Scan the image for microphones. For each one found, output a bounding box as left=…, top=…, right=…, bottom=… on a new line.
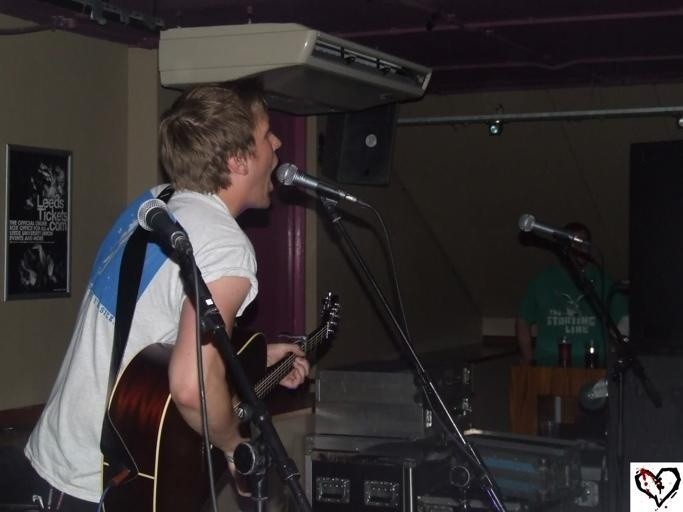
left=518, top=214, right=585, bottom=244
left=139, top=199, right=192, bottom=257
left=276, top=163, right=358, bottom=204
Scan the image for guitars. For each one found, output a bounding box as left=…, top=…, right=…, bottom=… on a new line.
left=99, top=289, right=342, bottom=511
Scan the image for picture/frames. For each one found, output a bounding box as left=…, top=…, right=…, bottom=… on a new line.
left=2, top=141, right=73, bottom=305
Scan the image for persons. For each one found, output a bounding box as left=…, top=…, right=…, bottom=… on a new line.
left=515, top=220, right=629, bottom=366
left=23, top=85, right=312, bottom=512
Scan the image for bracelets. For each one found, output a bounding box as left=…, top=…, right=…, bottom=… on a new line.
left=227, top=455, right=234, bottom=464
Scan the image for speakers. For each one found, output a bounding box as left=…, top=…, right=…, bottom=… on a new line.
left=321, top=99, right=399, bottom=187
left=609, top=355, right=683, bottom=511
left=630, top=140, right=683, bottom=355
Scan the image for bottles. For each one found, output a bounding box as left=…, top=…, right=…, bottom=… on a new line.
left=584, top=337, right=599, bottom=368
left=558, top=338, right=570, bottom=365
